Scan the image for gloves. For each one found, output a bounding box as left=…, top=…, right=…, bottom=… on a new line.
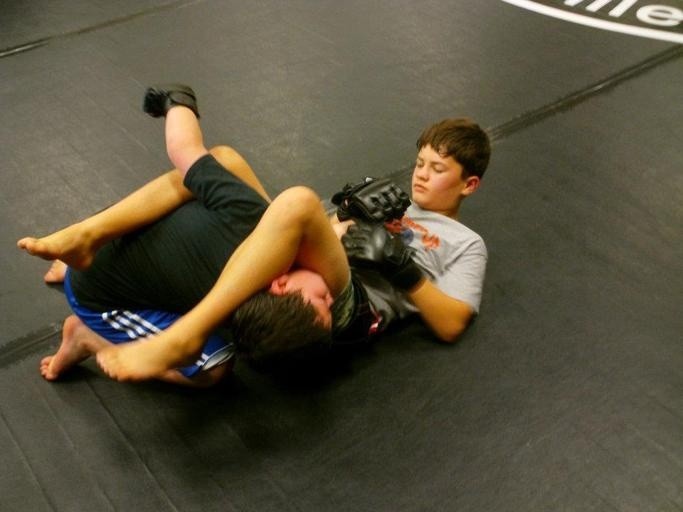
left=331, top=176, right=411, bottom=224
left=143, top=83, right=200, bottom=119
left=341, top=221, right=422, bottom=290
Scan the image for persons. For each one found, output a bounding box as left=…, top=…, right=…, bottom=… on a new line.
left=39, top=82, right=336, bottom=396
left=17, top=115, right=490, bottom=385
